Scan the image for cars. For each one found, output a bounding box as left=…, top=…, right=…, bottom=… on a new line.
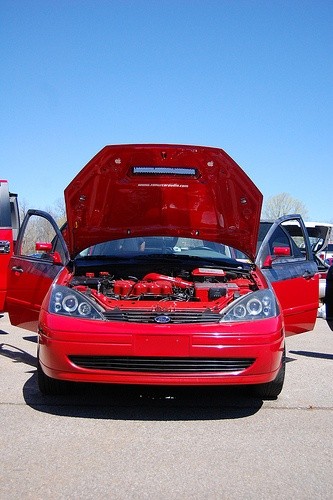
left=5, top=143, right=319, bottom=401
left=278, top=220, right=333, bottom=297
